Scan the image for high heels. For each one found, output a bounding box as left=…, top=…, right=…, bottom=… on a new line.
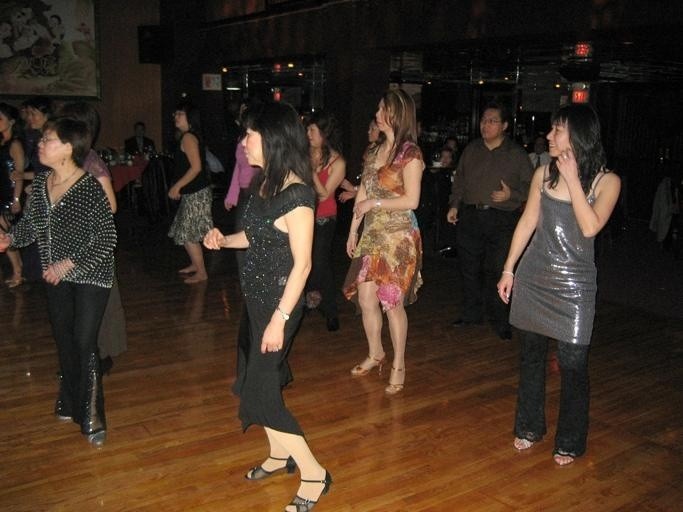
left=283, top=467, right=334, bottom=512
left=383, top=364, right=407, bottom=396
left=101, top=356, right=113, bottom=377
left=244, top=455, right=296, bottom=481
left=348, top=352, right=388, bottom=379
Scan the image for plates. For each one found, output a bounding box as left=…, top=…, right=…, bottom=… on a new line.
left=432, top=165, right=442, bottom=168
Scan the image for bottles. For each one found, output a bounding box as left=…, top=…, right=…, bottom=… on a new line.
left=516, top=123, right=528, bottom=142
left=419, top=115, right=470, bottom=146
left=665, top=148, right=670, bottom=161
left=97, top=145, right=153, bottom=168
left=656, top=147, right=665, bottom=168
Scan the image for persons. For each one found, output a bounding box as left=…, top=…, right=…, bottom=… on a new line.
left=299, top=95, right=386, bottom=332
left=160, top=100, right=262, bottom=294
left=343, top=89, right=428, bottom=395
left=1, top=117, right=117, bottom=447
left=50, top=99, right=127, bottom=377
left=0, top=99, right=50, bottom=288
left=125, top=123, right=156, bottom=207
left=497, top=105, right=621, bottom=464
left=416, top=106, right=553, bottom=342
left=204, top=99, right=332, bottom=511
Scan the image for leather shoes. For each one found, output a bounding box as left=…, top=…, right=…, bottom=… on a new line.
left=450, top=319, right=481, bottom=329
left=82, top=429, right=107, bottom=448
left=325, top=317, right=340, bottom=332
left=494, top=326, right=512, bottom=343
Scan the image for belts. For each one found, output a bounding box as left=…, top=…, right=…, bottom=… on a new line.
left=460, top=202, right=496, bottom=212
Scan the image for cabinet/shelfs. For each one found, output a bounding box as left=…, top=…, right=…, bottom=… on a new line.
left=224, top=54, right=327, bottom=138
left=391, top=41, right=579, bottom=151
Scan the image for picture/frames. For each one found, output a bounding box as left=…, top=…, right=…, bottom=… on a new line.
left=0, top=1, right=101, bottom=100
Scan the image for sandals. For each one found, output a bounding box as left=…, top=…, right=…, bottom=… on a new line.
left=552, top=450, right=576, bottom=466
left=512, top=436, right=537, bottom=451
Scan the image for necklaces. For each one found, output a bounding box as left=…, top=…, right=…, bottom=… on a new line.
left=50, top=166, right=79, bottom=193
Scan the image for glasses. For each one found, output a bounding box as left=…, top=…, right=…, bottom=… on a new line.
left=479, top=116, right=507, bottom=124
left=37, top=136, right=71, bottom=144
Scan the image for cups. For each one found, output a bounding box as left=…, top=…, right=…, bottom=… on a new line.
left=432, top=162, right=441, bottom=166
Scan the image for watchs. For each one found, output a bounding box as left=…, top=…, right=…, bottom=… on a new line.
left=277, top=307, right=291, bottom=322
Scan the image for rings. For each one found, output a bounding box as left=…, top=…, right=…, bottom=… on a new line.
left=564, top=156, right=568, bottom=159
